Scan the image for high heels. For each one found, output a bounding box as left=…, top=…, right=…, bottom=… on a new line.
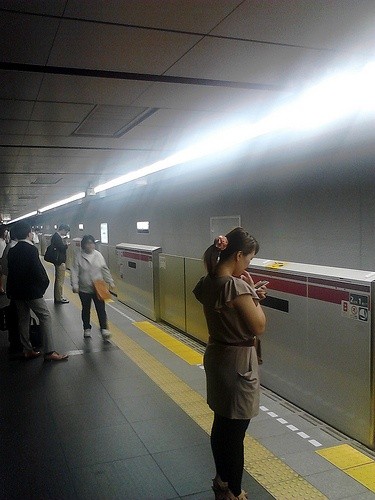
left=211, top=479, right=248, bottom=500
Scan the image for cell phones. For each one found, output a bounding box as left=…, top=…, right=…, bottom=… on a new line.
left=255, top=280, right=270, bottom=289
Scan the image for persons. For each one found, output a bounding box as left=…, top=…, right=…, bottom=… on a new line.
left=193, top=227, right=267, bottom=500
left=71, top=235, right=115, bottom=341
left=51, top=224, right=70, bottom=304
left=32, top=228, right=40, bottom=245
left=0, top=223, right=23, bottom=353
left=7, top=222, right=68, bottom=361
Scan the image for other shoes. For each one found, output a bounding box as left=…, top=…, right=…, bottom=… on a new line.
left=56, top=299, right=69, bottom=304
left=22, top=350, right=40, bottom=358
left=84, top=329, right=91, bottom=337
left=43, top=352, right=68, bottom=360
left=102, top=330, right=111, bottom=338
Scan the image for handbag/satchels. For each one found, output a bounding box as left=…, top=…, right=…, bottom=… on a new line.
left=29, top=317, right=42, bottom=347
left=92, top=280, right=111, bottom=300
left=44, top=237, right=63, bottom=265
